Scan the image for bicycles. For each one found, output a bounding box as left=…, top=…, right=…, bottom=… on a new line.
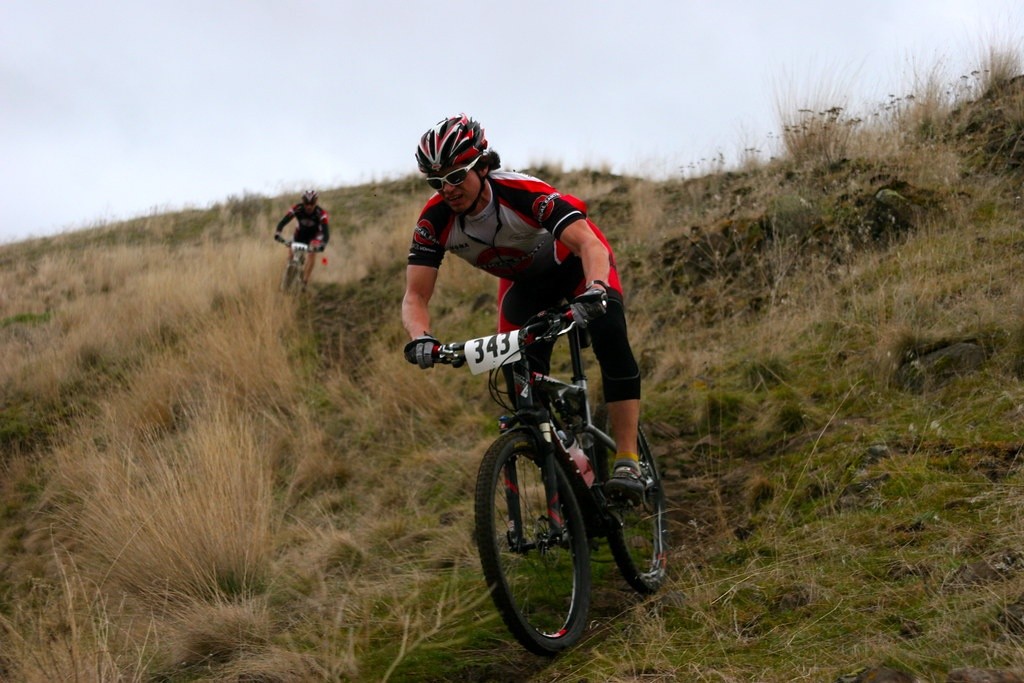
left=277, top=235, right=324, bottom=296
left=426, top=298, right=665, bottom=658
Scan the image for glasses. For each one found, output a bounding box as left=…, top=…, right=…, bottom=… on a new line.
left=304, top=202, right=314, bottom=207
left=426, top=155, right=482, bottom=190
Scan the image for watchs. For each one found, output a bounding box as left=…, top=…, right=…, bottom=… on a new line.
left=586, top=280, right=608, bottom=292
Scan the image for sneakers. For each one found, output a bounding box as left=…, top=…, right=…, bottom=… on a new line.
left=604, top=456, right=648, bottom=495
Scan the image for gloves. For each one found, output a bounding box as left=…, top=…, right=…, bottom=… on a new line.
left=570, top=279, right=606, bottom=329
left=318, top=244, right=324, bottom=252
left=403, top=329, right=440, bottom=369
left=274, top=234, right=280, bottom=240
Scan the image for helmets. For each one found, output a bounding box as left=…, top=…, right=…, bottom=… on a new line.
left=301, top=189, right=318, bottom=205
left=415, top=112, right=488, bottom=173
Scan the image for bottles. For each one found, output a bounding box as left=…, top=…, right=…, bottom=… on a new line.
left=555, top=429, right=595, bottom=489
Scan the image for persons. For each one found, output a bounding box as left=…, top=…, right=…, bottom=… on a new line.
left=274, top=190, right=330, bottom=291
left=402, top=114, right=647, bottom=508
